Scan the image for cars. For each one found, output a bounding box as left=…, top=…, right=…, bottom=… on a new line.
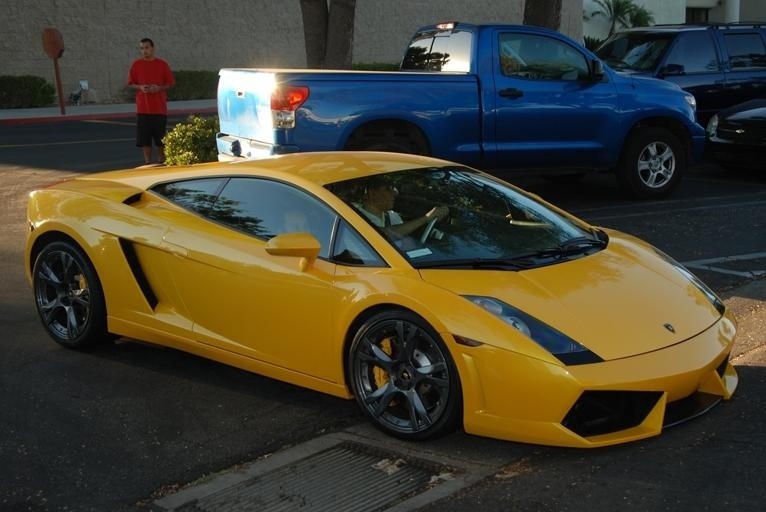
left=705, top=100, right=745, bottom=173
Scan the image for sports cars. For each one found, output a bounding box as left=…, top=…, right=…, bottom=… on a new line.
left=27, top=150, right=739, bottom=449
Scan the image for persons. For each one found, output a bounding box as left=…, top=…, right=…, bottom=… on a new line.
left=126, top=38, right=176, bottom=166
left=273, top=207, right=328, bottom=259
left=343, top=177, right=449, bottom=267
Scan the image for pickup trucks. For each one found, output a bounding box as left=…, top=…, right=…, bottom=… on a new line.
left=215, top=21, right=705, bottom=197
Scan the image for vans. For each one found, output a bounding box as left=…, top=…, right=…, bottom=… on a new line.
left=592, top=20, right=766, bottom=130
